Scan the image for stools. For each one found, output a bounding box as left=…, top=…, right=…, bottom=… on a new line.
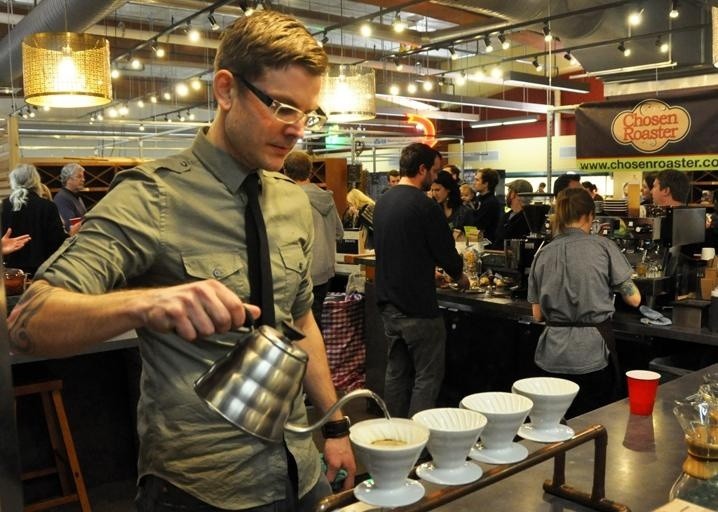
left=11, top=368, right=91, bottom=511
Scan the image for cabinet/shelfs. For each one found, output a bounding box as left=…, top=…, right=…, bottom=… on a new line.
left=640, top=157, right=718, bottom=229
left=23, top=156, right=138, bottom=212
left=311, top=156, right=368, bottom=221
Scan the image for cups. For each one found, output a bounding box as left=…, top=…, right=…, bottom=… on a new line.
left=414, top=406, right=483, bottom=473
left=513, top=376, right=580, bottom=432
left=626, top=368, right=661, bottom=417
left=347, top=416, right=423, bottom=491
left=68, top=218, right=81, bottom=224
left=462, top=390, right=529, bottom=450
left=637, top=262, right=648, bottom=278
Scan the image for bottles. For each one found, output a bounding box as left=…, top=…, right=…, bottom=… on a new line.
left=628, top=174, right=640, bottom=218
left=626, top=221, right=635, bottom=253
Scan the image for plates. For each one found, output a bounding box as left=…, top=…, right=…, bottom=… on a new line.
left=416, top=460, right=484, bottom=486
left=351, top=479, right=426, bottom=507
left=470, top=444, right=527, bottom=465
left=448, top=282, right=486, bottom=295
left=514, top=424, right=575, bottom=443
left=601, top=200, right=628, bottom=216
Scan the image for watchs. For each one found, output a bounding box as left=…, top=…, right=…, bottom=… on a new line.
left=322, top=415, right=351, bottom=438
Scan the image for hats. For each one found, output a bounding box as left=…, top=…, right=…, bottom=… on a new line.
left=503, top=179, right=533, bottom=193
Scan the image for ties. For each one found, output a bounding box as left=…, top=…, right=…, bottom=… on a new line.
left=239, top=172, right=277, bottom=334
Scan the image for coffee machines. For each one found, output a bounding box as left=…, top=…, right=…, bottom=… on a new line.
left=503, top=191, right=557, bottom=298
left=534, top=207, right=706, bottom=314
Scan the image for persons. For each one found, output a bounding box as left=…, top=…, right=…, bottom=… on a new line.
left=553, top=174, right=583, bottom=198
left=373, top=143, right=470, bottom=419
left=284, top=151, right=344, bottom=333
left=641, top=176, right=654, bottom=204
left=535, top=183, right=546, bottom=193
left=581, top=182, right=603, bottom=201
left=8, top=10, right=357, bottom=511
left=650, top=169, right=690, bottom=207
left=0, top=163, right=84, bottom=275
left=428, top=163, right=500, bottom=247
left=39, top=183, right=52, bottom=200
left=387, top=169, right=401, bottom=186
left=347, top=189, right=376, bottom=251
left=492, top=179, right=551, bottom=249
left=527, top=188, right=642, bottom=416
left=53, top=163, right=87, bottom=235
left=1, top=226, right=32, bottom=256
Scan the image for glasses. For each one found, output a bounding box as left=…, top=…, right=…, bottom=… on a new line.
left=230, top=70, right=327, bottom=132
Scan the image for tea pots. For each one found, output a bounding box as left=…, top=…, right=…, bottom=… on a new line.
left=191, top=308, right=373, bottom=446
